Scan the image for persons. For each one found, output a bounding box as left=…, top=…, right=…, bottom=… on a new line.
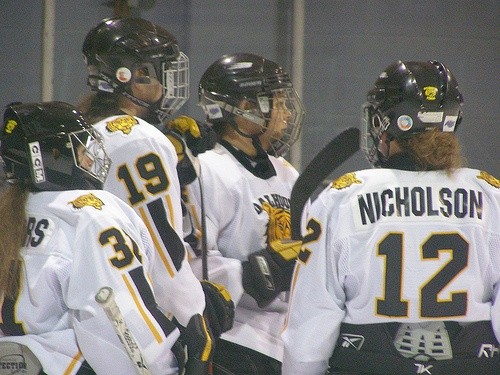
left=183, top=52, right=311, bottom=375
left=0, top=101, right=235, bottom=375
left=74, top=16, right=217, bottom=375
left=280, top=62, right=500, bottom=375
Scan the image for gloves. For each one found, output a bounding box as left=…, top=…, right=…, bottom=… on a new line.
left=182, top=314, right=216, bottom=375
left=241, top=238, right=305, bottom=309
left=200, top=279, right=235, bottom=337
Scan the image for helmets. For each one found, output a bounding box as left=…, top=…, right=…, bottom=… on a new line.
left=80, top=16, right=182, bottom=94
left=199, top=53, right=294, bottom=125
left=0, top=100, right=112, bottom=192
left=365, top=59, right=465, bottom=142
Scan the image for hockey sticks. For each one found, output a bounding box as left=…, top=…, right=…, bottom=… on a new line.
left=289, top=126, right=364, bottom=240
left=185, top=146, right=213, bottom=375
